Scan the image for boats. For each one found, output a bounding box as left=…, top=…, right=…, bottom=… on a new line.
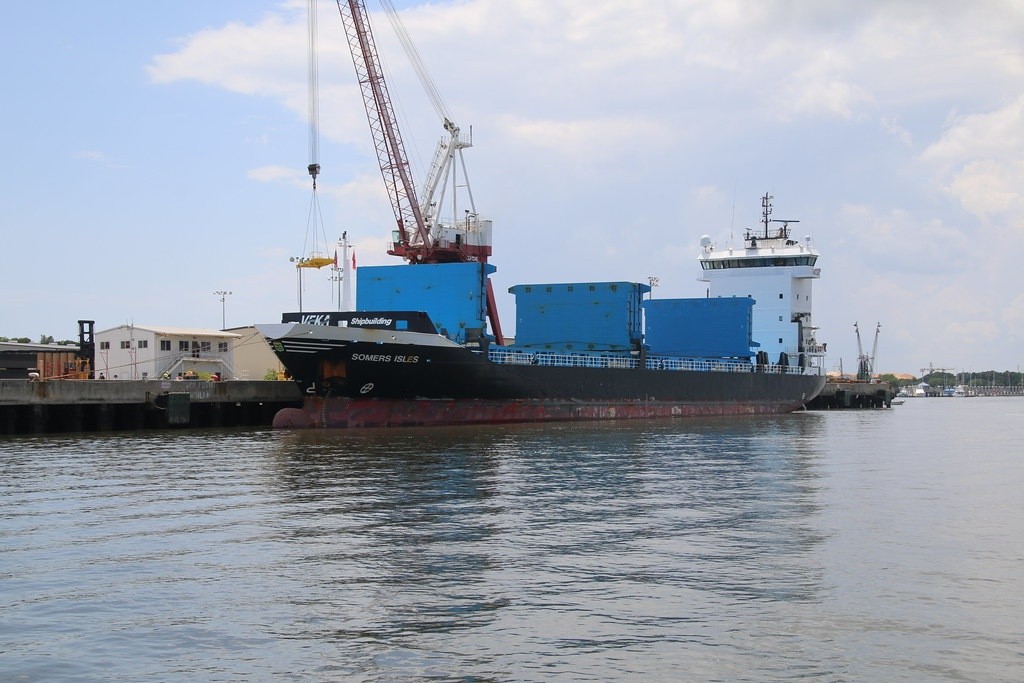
left=895, top=385, right=975, bottom=397
left=883, top=400, right=905, bottom=406
left=253, top=115, right=829, bottom=430
left=795, top=354, right=903, bottom=411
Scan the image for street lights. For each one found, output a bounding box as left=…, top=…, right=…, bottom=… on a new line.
left=334, top=267, right=344, bottom=313
left=214, top=290, right=232, bottom=330
left=290, top=257, right=311, bottom=324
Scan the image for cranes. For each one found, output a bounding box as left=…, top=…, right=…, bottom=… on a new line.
left=919, top=362, right=955, bottom=374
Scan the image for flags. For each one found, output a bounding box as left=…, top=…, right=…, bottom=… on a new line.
left=351, top=251, right=356, bottom=271
left=333, top=250, right=338, bottom=269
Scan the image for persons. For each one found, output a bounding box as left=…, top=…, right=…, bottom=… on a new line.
left=98, top=373, right=105, bottom=380
